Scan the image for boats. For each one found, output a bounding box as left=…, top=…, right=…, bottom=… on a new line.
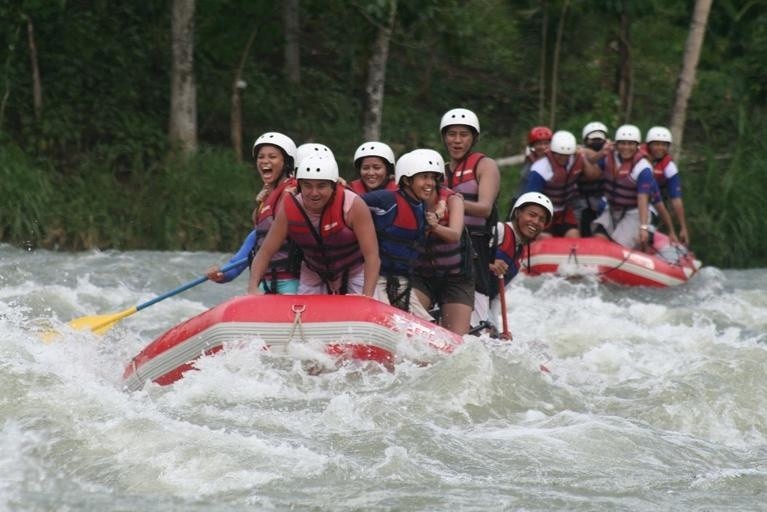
left=517, top=232, right=702, bottom=288
left=119, top=294, right=550, bottom=395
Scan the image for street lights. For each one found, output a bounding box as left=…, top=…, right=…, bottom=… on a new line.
left=235, top=75, right=250, bottom=162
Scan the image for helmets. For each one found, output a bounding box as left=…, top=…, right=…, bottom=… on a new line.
left=508, top=191, right=553, bottom=231
left=352, top=141, right=445, bottom=186
left=644, top=127, right=672, bottom=145
left=439, top=108, right=479, bottom=147
left=549, top=129, right=577, bottom=156
left=581, top=121, right=607, bottom=141
left=528, top=126, right=551, bottom=144
left=614, top=124, right=641, bottom=145
left=251, top=131, right=339, bottom=184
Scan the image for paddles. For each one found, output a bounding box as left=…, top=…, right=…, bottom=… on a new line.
left=68, top=259, right=250, bottom=332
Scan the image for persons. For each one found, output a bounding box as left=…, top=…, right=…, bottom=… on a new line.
left=520, top=122, right=689, bottom=255
left=203, top=108, right=554, bottom=334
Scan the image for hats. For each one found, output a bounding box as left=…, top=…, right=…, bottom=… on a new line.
left=587, top=131, right=606, bottom=140
